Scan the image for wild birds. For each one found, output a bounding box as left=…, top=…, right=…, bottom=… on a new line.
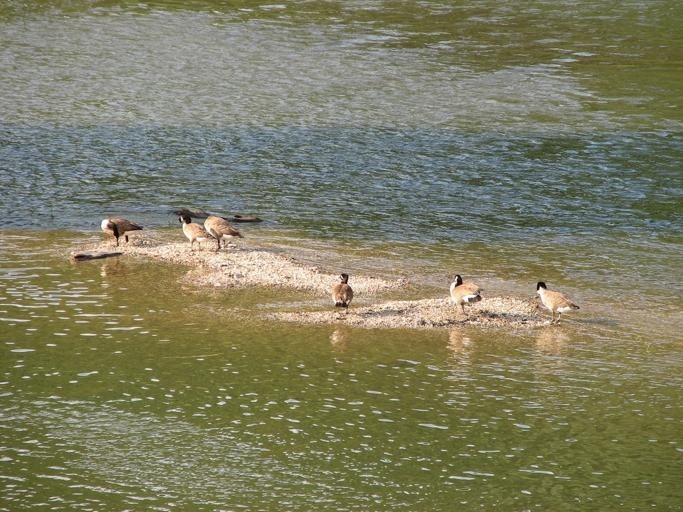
left=204, top=214, right=245, bottom=251
left=449, top=274, right=483, bottom=315
left=534, top=280, right=580, bottom=323
left=100, top=217, right=143, bottom=247
left=178, top=214, right=215, bottom=252
left=331, top=272, right=354, bottom=315
